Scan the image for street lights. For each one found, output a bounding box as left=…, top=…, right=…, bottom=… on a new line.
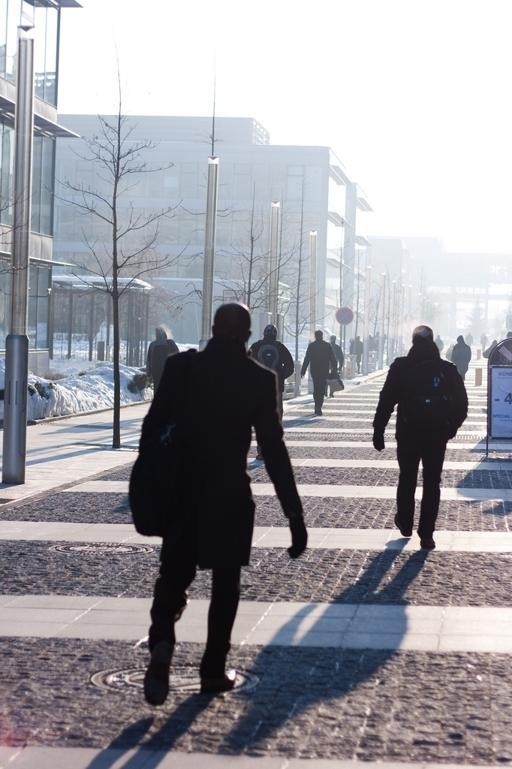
left=359, top=266, right=429, bottom=374
left=1, top=23, right=35, bottom=484
left=264, top=202, right=283, bottom=332
left=305, top=228, right=319, bottom=345
left=199, top=154, right=220, bottom=351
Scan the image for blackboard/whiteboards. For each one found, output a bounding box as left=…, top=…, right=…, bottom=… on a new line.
left=487, top=364, right=512, bottom=440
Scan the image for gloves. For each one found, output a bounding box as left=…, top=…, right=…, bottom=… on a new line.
left=286, top=517, right=308, bottom=556
left=373, top=427, right=385, bottom=451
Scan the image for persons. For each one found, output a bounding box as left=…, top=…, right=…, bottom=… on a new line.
left=464, top=332, right=473, bottom=346
left=299, top=329, right=336, bottom=416
left=140, top=302, right=307, bottom=705
left=324, top=335, right=344, bottom=398
left=372, top=326, right=468, bottom=549
left=480, top=333, right=486, bottom=352
left=146, top=326, right=180, bottom=396
left=450, top=335, right=471, bottom=382
left=435, top=335, right=444, bottom=354
left=249, top=324, right=294, bottom=460
left=348, top=332, right=404, bottom=373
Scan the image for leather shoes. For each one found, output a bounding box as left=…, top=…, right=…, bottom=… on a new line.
left=204, top=668, right=237, bottom=692
left=144, top=638, right=173, bottom=705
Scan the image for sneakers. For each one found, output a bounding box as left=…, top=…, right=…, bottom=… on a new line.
left=420, top=539, right=435, bottom=548
left=395, top=513, right=411, bottom=536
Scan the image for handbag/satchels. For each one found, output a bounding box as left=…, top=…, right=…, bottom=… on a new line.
left=129, top=450, right=177, bottom=535
left=327, top=373, right=344, bottom=392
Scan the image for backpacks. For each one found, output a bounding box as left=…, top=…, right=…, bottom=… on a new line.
left=258, top=341, right=280, bottom=368
